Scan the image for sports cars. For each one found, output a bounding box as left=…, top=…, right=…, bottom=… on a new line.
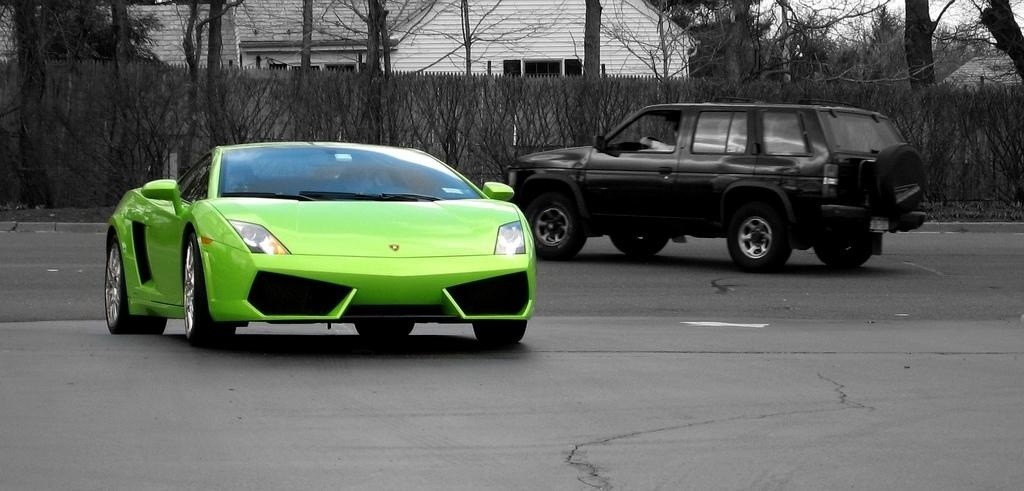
left=102, top=140, right=536, bottom=349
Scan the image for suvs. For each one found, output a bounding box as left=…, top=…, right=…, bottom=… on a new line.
left=507, top=101, right=926, bottom=275
